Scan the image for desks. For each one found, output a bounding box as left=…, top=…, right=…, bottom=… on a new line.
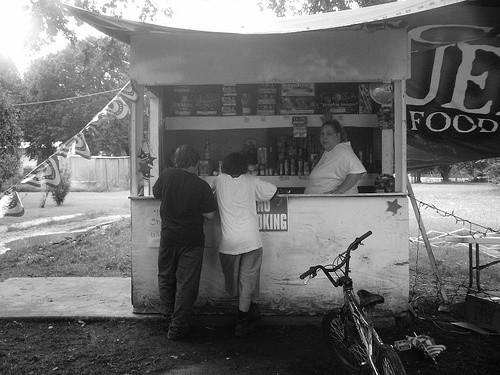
left=445, top=235, right=500, bottom=291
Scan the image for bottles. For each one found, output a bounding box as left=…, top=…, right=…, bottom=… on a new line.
left=202, top=136, right=214, bottom=163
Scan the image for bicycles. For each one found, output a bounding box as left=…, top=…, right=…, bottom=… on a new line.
left=300, top=231, right=411, bottom=375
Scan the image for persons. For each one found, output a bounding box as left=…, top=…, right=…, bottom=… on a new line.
left=303, top=119, right=367, bottom=194
left=211, top=152, right=279, bottom=332
left=151, top=144, right=216, bottom=336
left=36, top=139, right=60, bottom=207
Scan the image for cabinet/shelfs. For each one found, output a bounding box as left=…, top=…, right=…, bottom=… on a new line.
left=167, top=87, right=392, bottom=188
